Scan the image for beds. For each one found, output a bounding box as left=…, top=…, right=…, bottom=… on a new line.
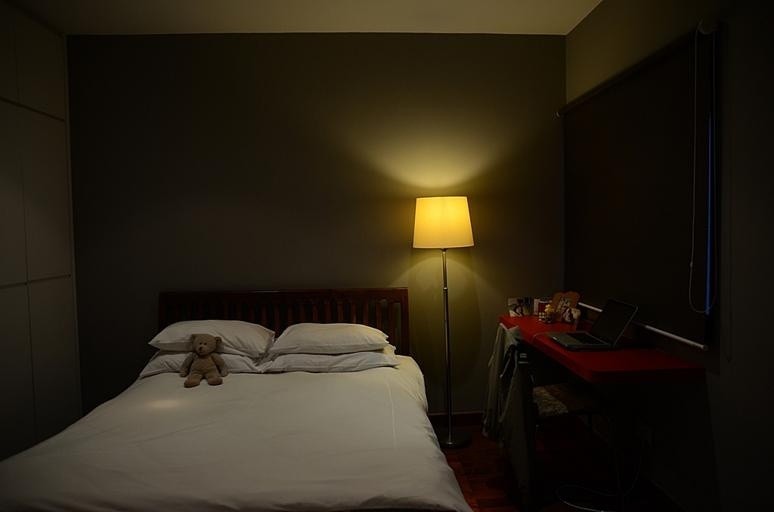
left=1, top=287, right=471, bottom=512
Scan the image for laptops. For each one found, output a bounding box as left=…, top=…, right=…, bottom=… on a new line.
left=545, top=298, right=639, bottom=350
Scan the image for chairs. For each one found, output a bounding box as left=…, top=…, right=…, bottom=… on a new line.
left=517, top=352, right=607, bottom=512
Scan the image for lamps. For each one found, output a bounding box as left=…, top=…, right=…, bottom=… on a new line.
left=412, top=197, right=477, bottom=451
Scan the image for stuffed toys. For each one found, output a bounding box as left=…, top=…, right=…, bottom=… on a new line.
left=179, top=333, right=228, bottom=387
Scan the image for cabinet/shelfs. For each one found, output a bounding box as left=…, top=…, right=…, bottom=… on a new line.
left=0, top=2, right=83, bottom=460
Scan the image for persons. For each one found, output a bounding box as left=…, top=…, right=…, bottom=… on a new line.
left=509, top=304, right=522, bottom=316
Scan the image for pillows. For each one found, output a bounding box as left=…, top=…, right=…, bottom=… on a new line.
left=140, top=319, right=396, bottom=382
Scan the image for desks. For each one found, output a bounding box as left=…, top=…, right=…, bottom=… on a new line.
left=496, top=315, right=707, bottom=384
